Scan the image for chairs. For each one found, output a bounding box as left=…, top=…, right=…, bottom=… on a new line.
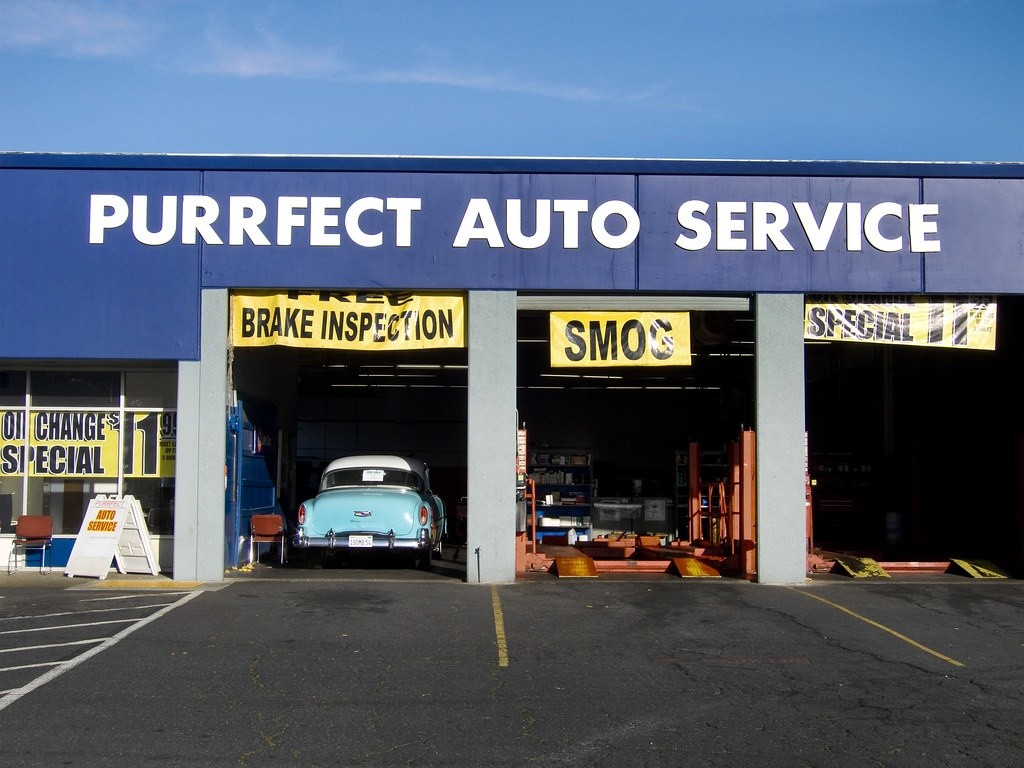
left=249, top=515, right=287, bottom=565
left=13, top=516, right=52, bottom=575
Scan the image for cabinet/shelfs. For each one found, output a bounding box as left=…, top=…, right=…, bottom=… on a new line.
left=675, top=450, right=728, bottom=528
left=527, top=448, right=594, bottom=541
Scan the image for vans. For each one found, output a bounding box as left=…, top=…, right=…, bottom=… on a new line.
left=291, top=455, right=447, bottom=571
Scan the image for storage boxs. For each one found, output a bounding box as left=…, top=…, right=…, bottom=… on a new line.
left=632, top=519, right=675, bottom=537
left=645, top=500, right=673, bottom=521
left=529, top=473, right=540, bottom=483
left=551, top=492, right=560, bottom=501
left=583, top=516, right=590, bottom=526
left=572, top=516, right=581, bottom=523
left=528, top=514, right=538, bottom=526
left=559, top=516, right=571, bottom=521
left=594, top=509, right=631, bottom=531
left=571, top=456, right=586, bottom=465
